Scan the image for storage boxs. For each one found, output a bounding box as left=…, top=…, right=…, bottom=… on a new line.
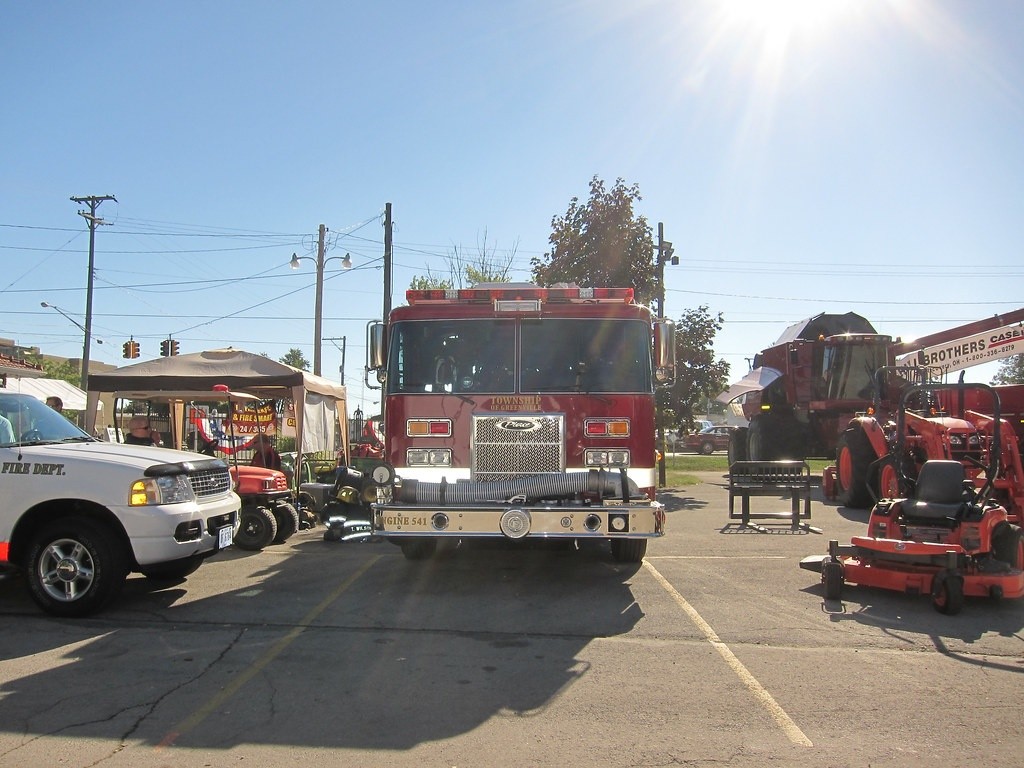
left=300, top=482, right=335, bottom=512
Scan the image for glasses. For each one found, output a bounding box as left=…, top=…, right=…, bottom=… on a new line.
left=47, top=404, right=59, bottom=407
left=253, top=441, right=258, bottom=444
left=133, top=426, right=149, bottom=431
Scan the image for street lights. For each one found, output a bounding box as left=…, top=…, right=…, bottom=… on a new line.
left=290, top=253, right=352, bottom=375
left=41, top=302, right=92, bottom=431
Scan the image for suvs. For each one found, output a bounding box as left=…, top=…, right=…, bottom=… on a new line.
left=655, top=421, right=714, bottom=453
left=0, top=391, right=242, bottom=618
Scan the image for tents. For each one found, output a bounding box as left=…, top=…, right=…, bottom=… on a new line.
left=84, top=347, right=351, bottom=532
left=0, top=378, right=104, bottom=442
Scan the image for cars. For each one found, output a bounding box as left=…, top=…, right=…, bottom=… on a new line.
left=686, top=426, right=739, bottom=455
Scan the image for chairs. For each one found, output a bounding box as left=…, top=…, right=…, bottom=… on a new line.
left=901, top=459, right=965, bottom=519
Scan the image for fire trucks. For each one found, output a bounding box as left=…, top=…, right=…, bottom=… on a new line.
left=364, top=283, right=677, bottom=560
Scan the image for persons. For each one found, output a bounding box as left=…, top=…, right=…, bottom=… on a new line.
left=33, top=396, right=63, bottom=441
left=249, top=433, right=280, bottom=473
left=123, top=416, right=161, bottom=447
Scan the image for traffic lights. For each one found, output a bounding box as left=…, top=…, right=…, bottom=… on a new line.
left=161, top=341, right=169, bottom=356
left=123, top=341, right=131, bottom=358
left=132, top=342, right=140, bottom=359
left=171, top=340, right=180, bottom=356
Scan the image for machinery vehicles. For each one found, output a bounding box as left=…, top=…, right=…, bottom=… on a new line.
left=714, top=312, right=1024, bottom=459
left=800, top=381, right=1024, bottom=615
left=835, top=349, right=1024, bottom=508
